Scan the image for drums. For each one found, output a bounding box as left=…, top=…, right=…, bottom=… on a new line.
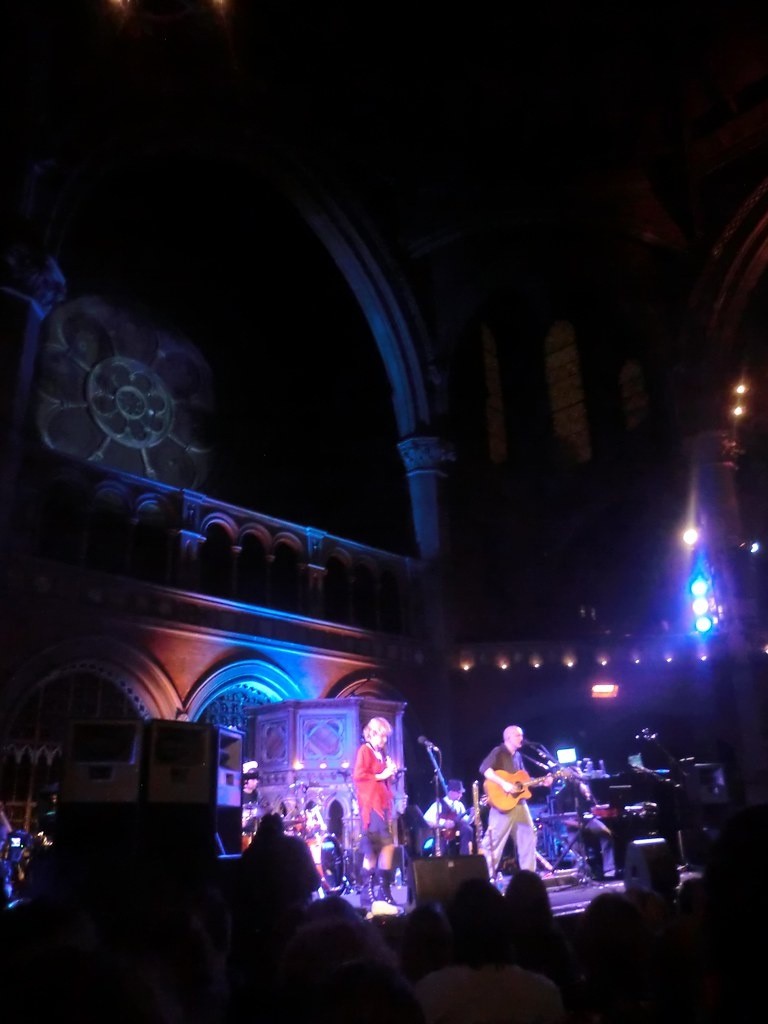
left=304, top=834, right=346, bottom=896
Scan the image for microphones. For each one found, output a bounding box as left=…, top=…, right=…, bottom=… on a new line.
left=417, top=736, right=439, bottom=752
left=520, top=740, right=540, bottom=746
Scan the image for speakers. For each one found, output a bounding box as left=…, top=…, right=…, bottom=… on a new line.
left=411, top=854, right=491, bottom=908
left=625, top=837, right=680, bottom=898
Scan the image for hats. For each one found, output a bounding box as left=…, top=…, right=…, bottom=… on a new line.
left=446, top=779, right=465, bottom=792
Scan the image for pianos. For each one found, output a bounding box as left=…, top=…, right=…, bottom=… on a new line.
left=593, top=805, right=643, bottom=817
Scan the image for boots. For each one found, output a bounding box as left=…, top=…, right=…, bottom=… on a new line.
left=360, top=867, right=377, bottom=911
left=376, top=869, right=405, bottom=914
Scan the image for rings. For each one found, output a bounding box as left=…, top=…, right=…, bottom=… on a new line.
left=393, top=766, right=395, bottom=769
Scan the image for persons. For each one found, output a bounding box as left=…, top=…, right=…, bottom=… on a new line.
left=479, top=725, right=553, bottom=877
left=559, top=766, right=617, bottom=877
left=423, top=780, right=475, bottom=855
left=0, top=803, right=768, bottom=1024
left=352, top=717, right=405, bottom=915
left=242, top=767, right=259, bottom=803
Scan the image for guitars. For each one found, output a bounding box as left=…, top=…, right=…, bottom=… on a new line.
left=482, top=768, right=561, bottom=813
left=440, top=801, right=481, bottom=841
